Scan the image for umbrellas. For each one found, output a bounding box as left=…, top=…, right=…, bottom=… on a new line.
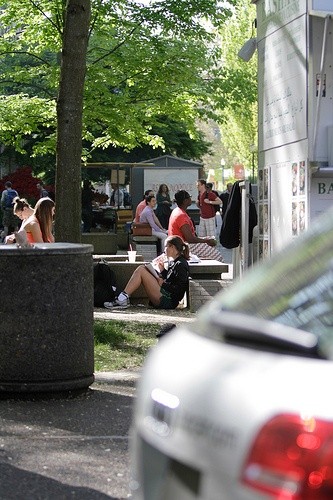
left=80, top=161, right=155, bottom=210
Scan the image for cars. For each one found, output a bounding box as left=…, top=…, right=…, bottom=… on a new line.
left=125, top=204, right=333, bottom=500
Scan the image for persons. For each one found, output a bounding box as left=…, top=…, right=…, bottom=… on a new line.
left=167, top=190, right=224, bottom=263
left=104, top=235, right=190, bottom=311
left=82, top=177, right=233, bottom=263
left=0, top=180, right=55, bottom=244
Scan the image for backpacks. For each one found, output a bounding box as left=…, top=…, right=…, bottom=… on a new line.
left=92, top=259, right=116, bottom=307
left=4, top=190, right=18, bottom=207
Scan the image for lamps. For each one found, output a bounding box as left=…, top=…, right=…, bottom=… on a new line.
left=237, top=19, right=258, bottom=63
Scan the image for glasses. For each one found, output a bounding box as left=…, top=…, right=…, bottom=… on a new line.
left=18, top=210, right=24, bottom=219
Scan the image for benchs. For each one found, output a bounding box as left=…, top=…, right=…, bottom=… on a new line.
left=129, top=235, right=158, bottom=261
left=188, top=258, right=229, bottom=309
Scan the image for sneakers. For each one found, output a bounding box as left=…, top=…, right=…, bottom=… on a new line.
left=104, top=298, right=130, bottom=309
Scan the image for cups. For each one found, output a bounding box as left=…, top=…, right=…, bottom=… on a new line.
left=152, top=252, right=166, bottom=264
left=127, top=251, right=137, bottom=262
